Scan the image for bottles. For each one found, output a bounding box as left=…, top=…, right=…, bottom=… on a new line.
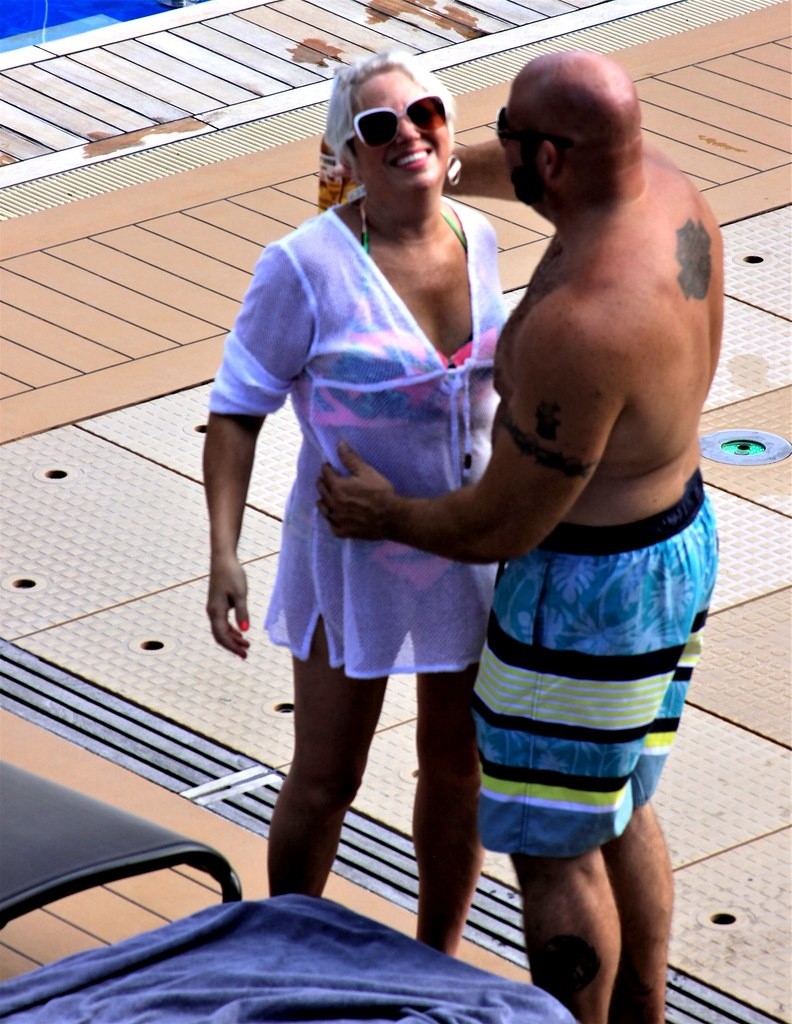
left=318, top=140, right=363, bottom=216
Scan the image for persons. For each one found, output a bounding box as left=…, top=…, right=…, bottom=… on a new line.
left=202, top=49, right=724, bottom=1023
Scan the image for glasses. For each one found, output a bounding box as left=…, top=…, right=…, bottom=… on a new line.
left=341, top=95, right=448, bottom=151
left=496, top=106, right=576, bottom=151
